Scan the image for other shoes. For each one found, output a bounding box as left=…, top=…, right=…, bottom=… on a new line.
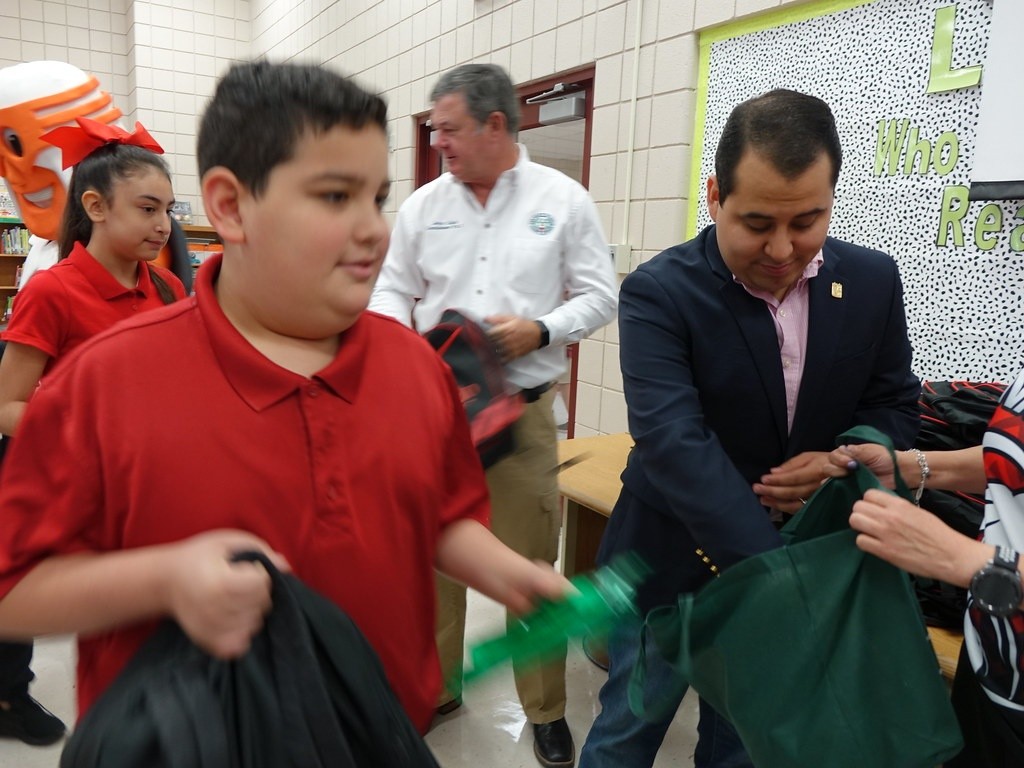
left=0, top=693, right=64, bottom=745
left=582, top=634, right=610, bottom=672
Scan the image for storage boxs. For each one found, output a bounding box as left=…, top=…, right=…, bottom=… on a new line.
left=184, top=241, right=223, bottom=268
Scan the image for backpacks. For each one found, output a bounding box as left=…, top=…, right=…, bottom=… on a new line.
left=419, top=311, right=525, bottom=469
left=57, top=550, right=440, bottom=768
left=916, top=377, right=1009, bottom=632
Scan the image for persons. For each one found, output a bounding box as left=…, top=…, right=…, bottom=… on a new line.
left=822, top=359, right=1023, bottom=768
left=0, top=60, right=193, bottom=304
left=577, top=89, right=920, bottom=768
left=0, top=141, right=189, bottom=437
left=0, top=340, right=65, bottom=747
left=0, top=59, right=582, bottom=740
left=364, top=63, right=619, bottom=768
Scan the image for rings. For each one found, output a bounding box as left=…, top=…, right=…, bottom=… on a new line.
left=799, top=497, right=808, bottom=504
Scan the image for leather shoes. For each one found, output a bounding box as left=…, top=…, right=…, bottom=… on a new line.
left=437, top=695, right=463, bottom=715
left=533, top=717, right=575, bottom=768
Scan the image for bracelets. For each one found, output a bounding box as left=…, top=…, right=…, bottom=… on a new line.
left=534, top=320, right=549, bottom=349
left=906, top=447, right=929, bottom=503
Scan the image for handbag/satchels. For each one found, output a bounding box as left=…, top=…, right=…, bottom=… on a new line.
left=624, top=463, right=961, bottom=768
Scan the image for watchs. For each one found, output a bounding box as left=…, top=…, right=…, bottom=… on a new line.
left=968, top=545, right=1022, bottom=617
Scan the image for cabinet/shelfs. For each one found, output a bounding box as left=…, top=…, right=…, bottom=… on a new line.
left=0, top=216, right=227, bottom=363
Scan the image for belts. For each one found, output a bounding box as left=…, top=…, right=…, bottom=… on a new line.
left=534, top=381, right=557, bottom=394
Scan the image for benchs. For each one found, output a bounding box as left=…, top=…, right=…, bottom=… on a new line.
left=556, top=435, right=963, bottom=688
